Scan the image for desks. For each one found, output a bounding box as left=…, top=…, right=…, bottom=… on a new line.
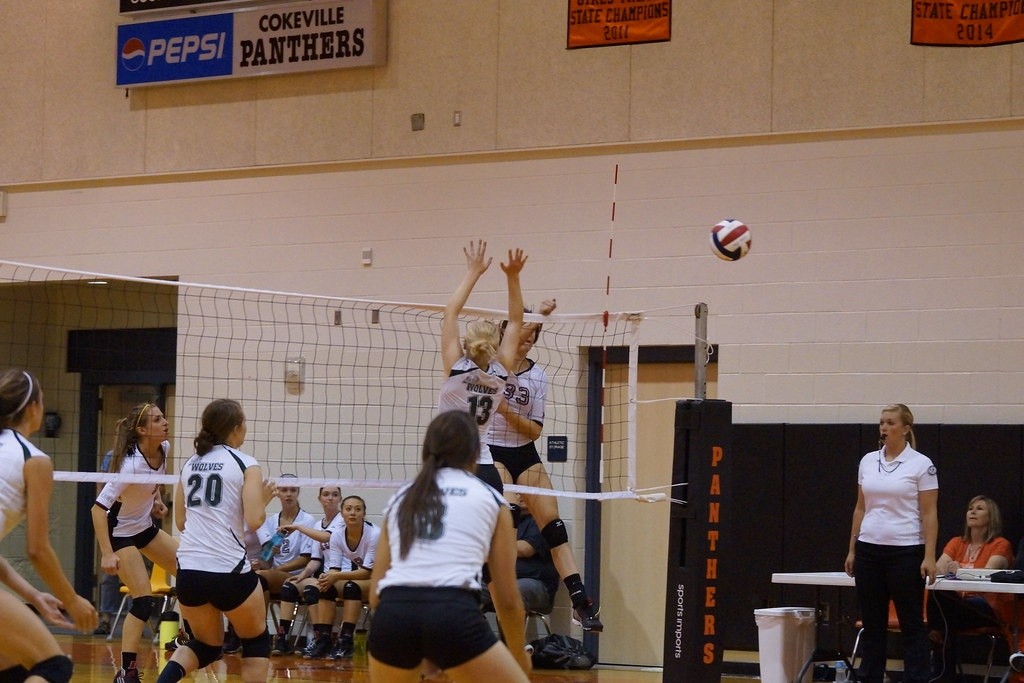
left=770, top=571, right=1024, bottom=683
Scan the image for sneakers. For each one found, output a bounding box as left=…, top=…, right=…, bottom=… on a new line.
left=222, top=636, right=242, bottom=653
left=272, top=641, right=286, bottom=656
left=94, top=622, right=111, bottom=635
left=165, top=634, right=189, bottom=650
left=327, top=641, right=354, bottom=659
left=302, top=640, right=331, bottom=658
left=113, top=666, right=144, bottom=683
left=572, top=605, right=603, bottom=634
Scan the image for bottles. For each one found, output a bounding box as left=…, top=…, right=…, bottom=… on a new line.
left=352, top=629, right=367, bottom=666
left=260, top=528, right=287, bottom=562
left=835, top=661, right=847, bottom=683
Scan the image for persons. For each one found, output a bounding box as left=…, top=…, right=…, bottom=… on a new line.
left=155, top=399, right=280, bottom=683
left=929, top=495, right=1015, bottom=683
left=224, top=474, right=381, bottom=660
left=418, top=239, right=531, bottom=681
left=0, top=368, right=98, bottom=683
left=91, top=404, right=179, bottom=683
left=366, top=411, right=532, bottom=683
left=486, top=299, right=604, bottom=634
left=482, top=493, right=560, bottom=614
left=845, top=404, right=939, bottom=683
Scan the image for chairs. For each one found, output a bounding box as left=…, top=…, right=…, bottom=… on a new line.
left=105, top=561, right=173, bottom=644
left=845, top=587, right=929, bottom=680
left=269, top=593, right=372, bottom=646
left=950, top=592, right=1015, bottom=683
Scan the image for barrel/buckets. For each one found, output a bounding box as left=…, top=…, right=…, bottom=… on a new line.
left=155, top=611, right=179, bottom=648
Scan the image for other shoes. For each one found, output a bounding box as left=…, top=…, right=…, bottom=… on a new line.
left=928, top=630, right=949, bottom=653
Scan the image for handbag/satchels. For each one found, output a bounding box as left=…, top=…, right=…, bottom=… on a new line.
left=529, top=635, right=595, bottom=669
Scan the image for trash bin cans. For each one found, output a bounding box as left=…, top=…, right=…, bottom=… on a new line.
left=753, top=607, right=817, bottom=683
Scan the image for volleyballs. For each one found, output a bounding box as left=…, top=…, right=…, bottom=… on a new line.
left=709, top=218, right=752, bottom=261
